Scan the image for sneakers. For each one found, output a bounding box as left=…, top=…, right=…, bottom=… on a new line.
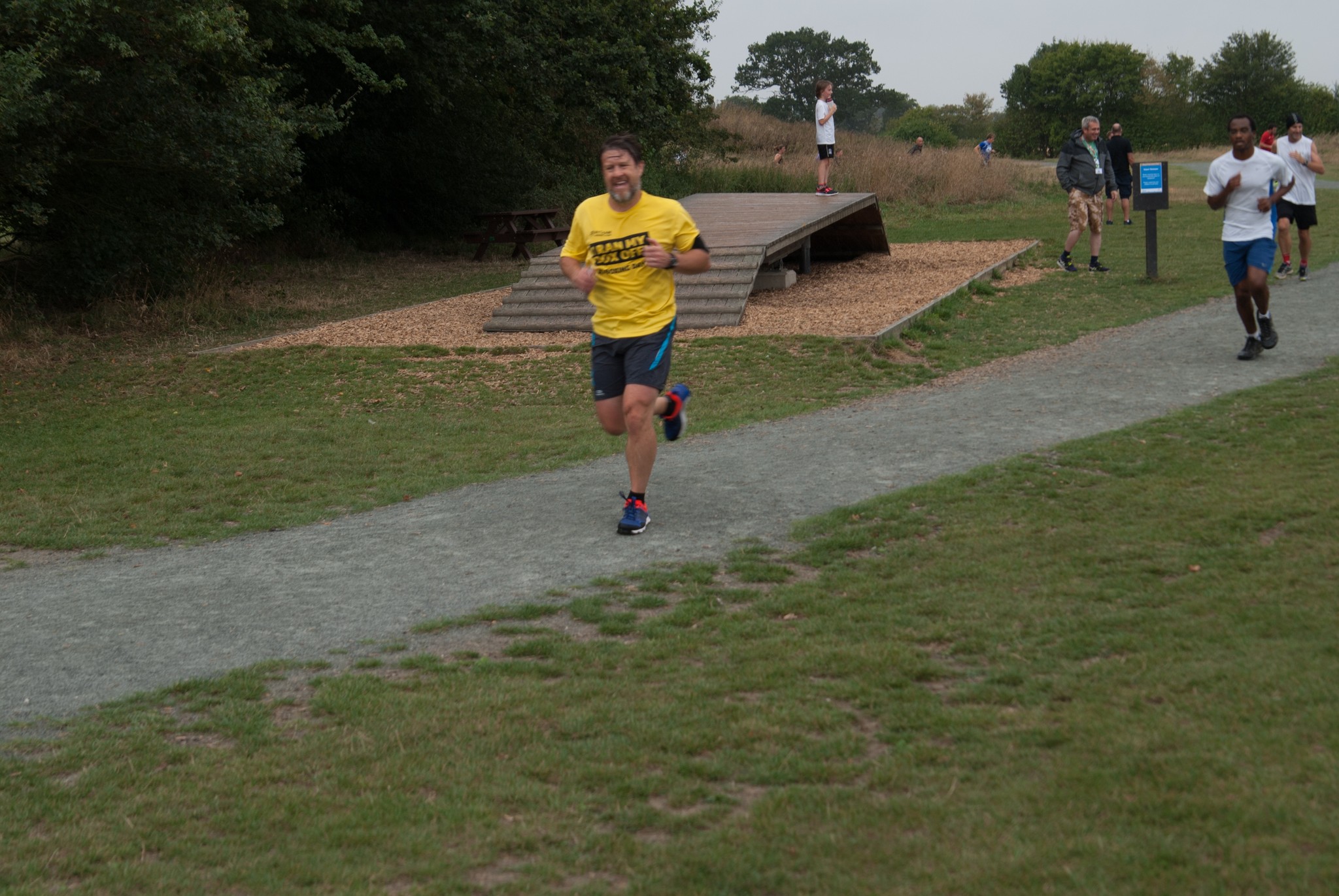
left=815, top=187, right=835, bottom=196
left=1257, top=310, right=1278, bottom=349
left=1273, top=263, right=1293, bottom=279
left=1057, top=256, right=1077, bottom=273
left=662, top=383, right=690, bottom=440
left=1299, top=266, right=1308, bottom=281
left=1237, top=335, right=1264, bottom=359
left=827, top=185, right=838, bottom=194
left=1089, top=262, right=1109, bottom=272
left=616, top=491, right=650, bottom=534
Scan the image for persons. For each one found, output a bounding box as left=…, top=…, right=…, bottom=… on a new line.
left=816, top=80, right=839, bottom=196
left=1057, top=116, right=1135, bottom=273
left=561, top=134, right=712, bottom=536
left=775, top=145, right=785, bottom=164
left=906, top=136, right=924, bottom=153
left=974, top=134, right=996, bottom=167
left=1204, top=113, right=1325, bottom=361
left=817, top=148, right=844, bottom=162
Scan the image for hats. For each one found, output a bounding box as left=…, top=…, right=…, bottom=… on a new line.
left=1285, top=113, right=1302, bottom=130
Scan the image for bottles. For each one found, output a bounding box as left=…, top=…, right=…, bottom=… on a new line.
left=827, top=98, right=834, bottom=108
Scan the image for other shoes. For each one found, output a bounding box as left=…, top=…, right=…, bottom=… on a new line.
left=1107, top=220, right=1113, bottom=224
left=1124, top=220, right=1133, bottom=225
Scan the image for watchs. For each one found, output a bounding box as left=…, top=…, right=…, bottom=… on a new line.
left=1301, top=158, right=1309, bottom=166
left=665, top=253, right=679, bottom=269
